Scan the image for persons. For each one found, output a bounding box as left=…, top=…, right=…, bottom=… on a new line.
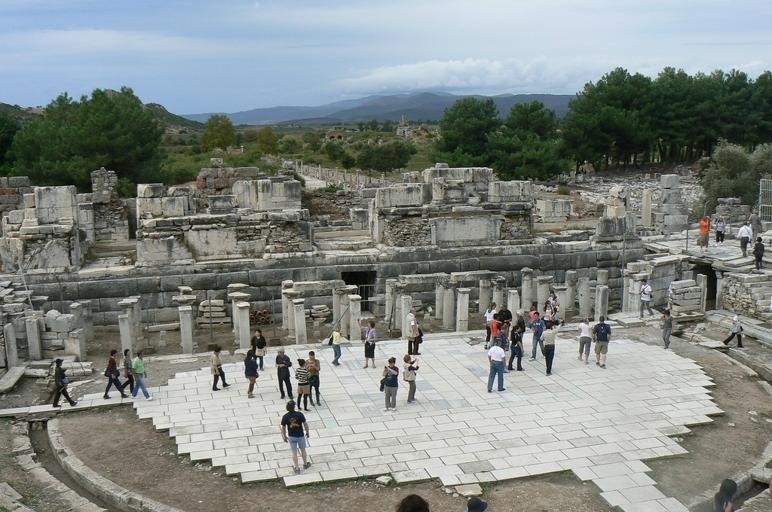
left=212, top=306, right=423, bottom=473
left=121, top=349, right=134, bottom=393
left=699, top=209, right=764, bottom=270
left=724, top=310, right=745, bottom=349
left=463, top=498, right=487, bottom=512
left=132, top=350, right=155, bottom=401
left=639, top=279, right=655, bottom=319
left=396, top=495, right=429, bottom=512
left=661, top=309, right=673, bottom=350
left=52, top=359, right=77, bottom=407
left=103, top=349, right=129, bottom=398
left=482, top=286, right=610, bottom=395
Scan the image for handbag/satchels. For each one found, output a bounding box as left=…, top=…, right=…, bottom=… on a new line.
left=380, top=378, right=385, bottom=391
left=256, top=349, right=265, bottom=356
left=329, top=337, right=333, bottom=345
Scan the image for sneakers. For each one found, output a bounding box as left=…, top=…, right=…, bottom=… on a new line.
left=303, top=463, right=310, bottom=469
left=293, top=466, right=300, bottom=473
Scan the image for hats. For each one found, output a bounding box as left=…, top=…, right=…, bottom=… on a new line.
left=56, top=359, right=63, bottom=364
left=469, top=498, right=487, bottom=512
